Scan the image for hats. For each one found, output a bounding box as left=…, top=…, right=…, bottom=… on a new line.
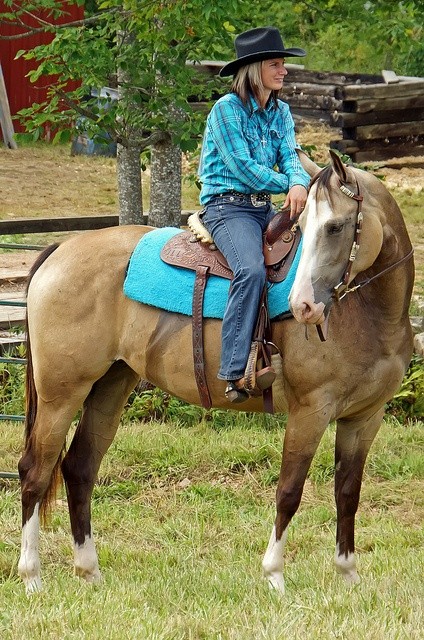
left=219, top=27, right=307, bottom=78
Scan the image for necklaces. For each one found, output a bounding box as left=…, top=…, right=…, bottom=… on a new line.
left=254, top=110, right=271, bottom=149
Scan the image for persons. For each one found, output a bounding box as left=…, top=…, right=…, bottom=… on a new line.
left=196, top=25, right=311, bottom=401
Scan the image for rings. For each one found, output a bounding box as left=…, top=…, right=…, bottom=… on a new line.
left=300, top=207, right=304, bottom=208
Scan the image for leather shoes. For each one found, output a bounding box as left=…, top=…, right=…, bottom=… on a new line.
left=226, top=366, right=276, bottom=403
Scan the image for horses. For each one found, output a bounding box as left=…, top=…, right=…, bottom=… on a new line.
left=17, top=147, right=414, bottom=599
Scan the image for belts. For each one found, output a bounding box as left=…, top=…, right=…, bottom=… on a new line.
left=215, top=192, right=271, bottom=209
